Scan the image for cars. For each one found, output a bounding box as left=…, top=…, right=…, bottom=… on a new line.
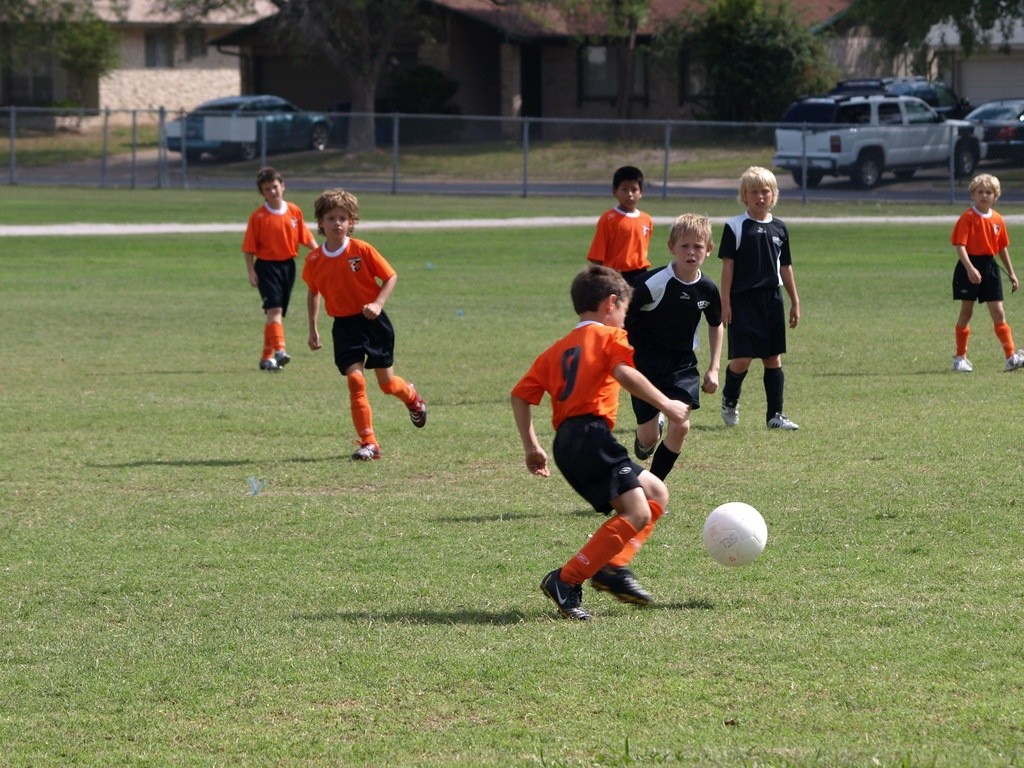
left=166, top=95, right=334, bottom=165
left=964, top=99, right=1024, bottom=158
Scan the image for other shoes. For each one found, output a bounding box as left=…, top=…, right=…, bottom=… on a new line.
left=275, top=352, right=290, bottom=367
left=259, top=359, right=282, bottom=371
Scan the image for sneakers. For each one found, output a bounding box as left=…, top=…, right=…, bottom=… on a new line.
left=1006, top=350, right=1024, bottom=371
left=721, top=384, right=739, bottom=426
left=951, top=356, right=972, bottom=371
left=540, top=568, right=591, bottom=621
left=351, top=439, right=380, bottom=459
left=408, top=395, right=427, bottom=428
left=633, top=412, right=665, bottom=461
left=591, top=564, right=651, bottom=605
left=766, top=412, right=799, bottom=430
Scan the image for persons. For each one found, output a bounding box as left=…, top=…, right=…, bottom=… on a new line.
left=950, top=173, right=1024, bottom=373
left=240, top=167, right=320, bottom=371
left=303, top=188, right=426, bottom=462
left=622, top=214, right=722, bottom=480
left=510, top=265, right=690, bottom=620
left=587, top=166, right=653, bottom=288
left=717, top=166, right=801, bottom=431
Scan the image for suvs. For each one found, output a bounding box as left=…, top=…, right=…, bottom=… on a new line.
left=828, top=75, right=977, bottom=121
left=771, top=93, right=988, bottom=191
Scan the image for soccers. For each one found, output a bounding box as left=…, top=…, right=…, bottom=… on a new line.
left=702, top=502, right=768, bottom=567
